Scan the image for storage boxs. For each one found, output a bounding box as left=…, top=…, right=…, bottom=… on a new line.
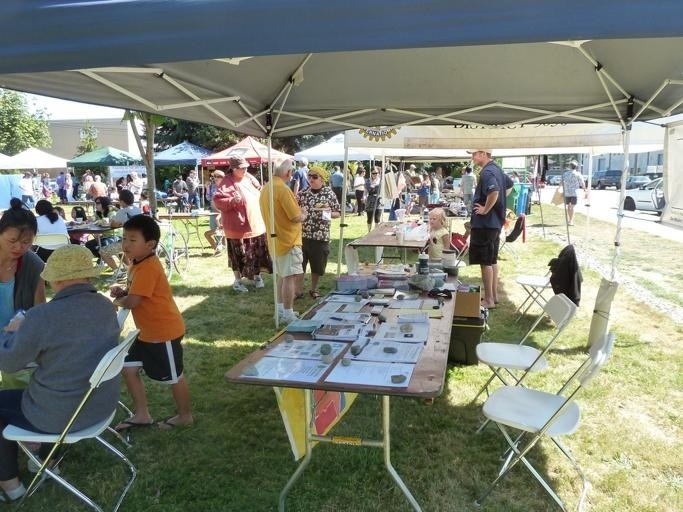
left=448, top=318, right=483, bottom=365
left=454, top=285, right=482, bottom=317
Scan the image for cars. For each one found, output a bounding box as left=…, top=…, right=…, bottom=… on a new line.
left=624, top=177, right=674, bottom=217
left=541, top=174, right=587, bottom=186
left=626, top=175, right=651, bottom=188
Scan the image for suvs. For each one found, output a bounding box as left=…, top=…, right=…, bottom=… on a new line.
left=584, top=169, right=624, bottom=189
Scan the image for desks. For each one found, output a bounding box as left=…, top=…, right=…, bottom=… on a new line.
left=158, top=212, right=220, bottom=257
left=347, top=215, right=431, bottom=265
left=223, top=264, right=458, bottom=512
left=67, top=223, right=115, bottom=265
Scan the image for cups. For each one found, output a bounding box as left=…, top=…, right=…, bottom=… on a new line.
left=396, top=231, right=403, bottom=244
left=395, top=209, right=406, bottom=221
left=83, top=198, right=86, bottom=201
left=102, top=218, right=110, bottom=224
left=76, top=218, right=83, bottom=222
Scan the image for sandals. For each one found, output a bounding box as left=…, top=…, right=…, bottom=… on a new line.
left=310, top=290, right=322, bottom=299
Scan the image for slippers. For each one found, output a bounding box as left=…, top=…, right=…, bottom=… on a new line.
left=115, top=420, right=154, bottom=432
left=157, top=417, right=175, bottom=429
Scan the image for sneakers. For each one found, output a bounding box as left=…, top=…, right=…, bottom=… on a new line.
left=27, top=455, right=60, bottom=479
left=233, top=282, right=248, bottom=292
left=252, top=278, right=264, bottom=288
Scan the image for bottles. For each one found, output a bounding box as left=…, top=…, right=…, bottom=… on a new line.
left=441, top=250, right=457, bottom=269
left=418, top=251, right=430, bottom=276
left=424, top=208, right=429, bottom=222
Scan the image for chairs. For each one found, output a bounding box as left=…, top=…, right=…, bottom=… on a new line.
left=1, top=327, right=141, bottom=512
left=472, top=291, right=577, bottom=435
left=33, top=233, right=69, bottom=251
left=116, top=306, right=130, bottom=329
left=513, top=244, right=575, bottom=323
left=471, top=329, right=617, bottom=512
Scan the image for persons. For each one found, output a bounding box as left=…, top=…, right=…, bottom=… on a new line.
left=294, top=167, right=342, bottom=300
left=561, top=159, right=588, bottom=226
left=405, top=163, right=476, bottom=217
left=0, top=245, right=122, bottom=503
left=332, top=165, right=382, bottom=231
left=294, top=162, right=306, bottom=192
left=213, top=157, right=273, bottom=291
left=466, top=149, right=520, bottom=309
left=0, top=198, right=47, bottom=333
left=259, top=158, right=306, bottom=327
left=10, top=169, right=148, bottom=286
left=110, top=215, right=195, bottom=433
left=173, top=169, right=226, bottom=256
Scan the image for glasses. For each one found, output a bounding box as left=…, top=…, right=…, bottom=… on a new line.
left=308, top=174, right=318, bottom=179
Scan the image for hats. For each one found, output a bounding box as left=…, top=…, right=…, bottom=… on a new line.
left=40, top=244, right=96, bottom=282
left=230, top=156, right=250, bottom=168
left=466, top=148, right=492, bottom=154
